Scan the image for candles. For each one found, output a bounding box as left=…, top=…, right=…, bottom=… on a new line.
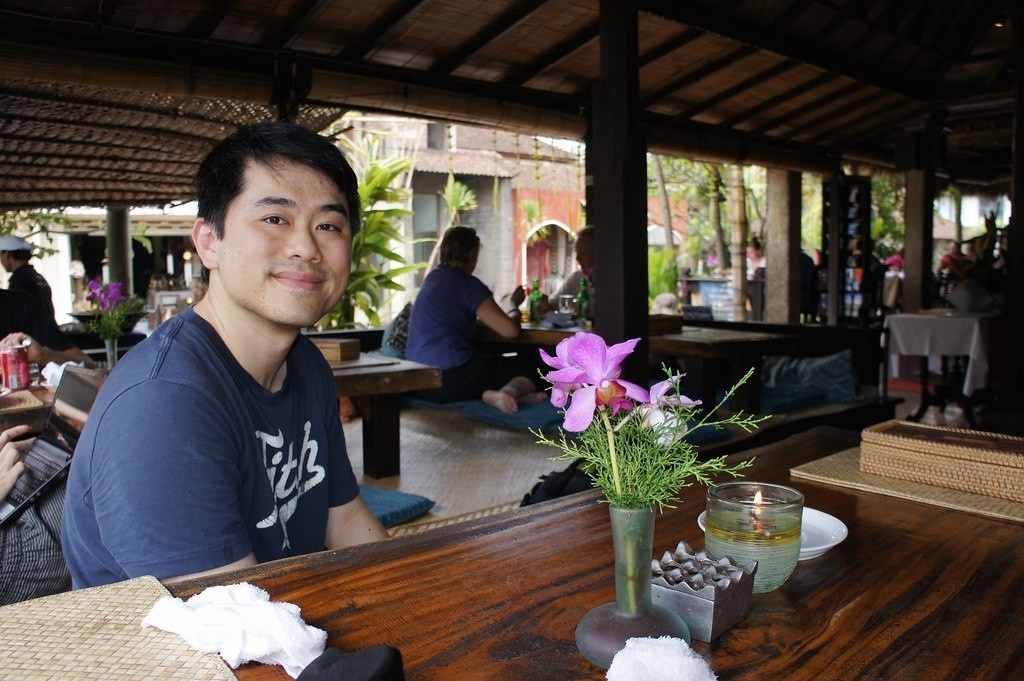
left=704, top=482, right=806, bottom=596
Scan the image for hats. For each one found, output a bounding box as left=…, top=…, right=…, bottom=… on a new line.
left=0, top=235, right=31, bottom=251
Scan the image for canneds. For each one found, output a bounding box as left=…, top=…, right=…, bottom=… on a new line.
left=0, top=344, right=30, bottom=391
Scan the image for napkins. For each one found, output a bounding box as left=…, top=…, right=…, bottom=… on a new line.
left=140, top=580, right=330, bottom=679
left=40, top=360, right=85, bottom=385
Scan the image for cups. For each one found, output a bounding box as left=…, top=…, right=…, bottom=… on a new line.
left=706, top=481, right=804, bottom=593
left=28, top=363, right=39, bottom=387
left=559, top=295, right=574, bottom=314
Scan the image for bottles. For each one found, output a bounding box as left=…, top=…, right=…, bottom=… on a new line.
left=529, top=279, right=542, bottom=322
left=519, top=284, right=529, bottom=323
left=576, top=278, right=589, bottom=322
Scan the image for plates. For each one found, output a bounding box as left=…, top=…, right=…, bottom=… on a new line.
left=698, top=505, right=848, bottom=561
left=931, top=308, right=968, bottom=318
left=0, top=386, right=12, bottom=400
left=40, top=381, right=59, bottom=392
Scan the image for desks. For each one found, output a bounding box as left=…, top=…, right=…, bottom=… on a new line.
left=316, top=352, right=444, bottom=477
left=0, top=427, right=1024, bottom=681
left=0, top=387, right=54, bottom=432
left=688, top=321, right=847, bottom=409
left=479, top=324, right=780, bottom=425
left=880, top=308, right=1013, bottom=428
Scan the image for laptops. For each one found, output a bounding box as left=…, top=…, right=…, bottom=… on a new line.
left=0, top=365, right=109, bottom=529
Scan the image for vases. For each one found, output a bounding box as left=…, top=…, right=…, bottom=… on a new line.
left=573, top=500, right=695, bottom=669
left=103, top=340, right=118, bottom=370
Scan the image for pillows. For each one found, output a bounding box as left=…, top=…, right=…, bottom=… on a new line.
left=761, top=355, right=862, bottom=404
left=357, top=483, right=435, bottom=531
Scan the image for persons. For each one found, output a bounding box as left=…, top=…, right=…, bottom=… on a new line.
left=548, top=227, right=594, bottom=318
left=714, top=235, right=766, bottom=278
left=800, top=250, right=820, bottom=314
left=0, top=424, right=72, bottom=605
left=60, top=121, right=393, bottom=584
left=405, top=226, right=558, bottom=415
left=0, top=289, right=97, bottom=369
left=0, top=234, right=55, bottom=317
left=849, top=237, right=967, bottom=290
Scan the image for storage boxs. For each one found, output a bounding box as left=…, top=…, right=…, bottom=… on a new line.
left=309, top=338, right=360, bottom=362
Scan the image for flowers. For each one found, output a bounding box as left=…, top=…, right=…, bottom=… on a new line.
left=84, top=281, right=138, bottom=340
left=524, top=329, right=774, bottom=515
